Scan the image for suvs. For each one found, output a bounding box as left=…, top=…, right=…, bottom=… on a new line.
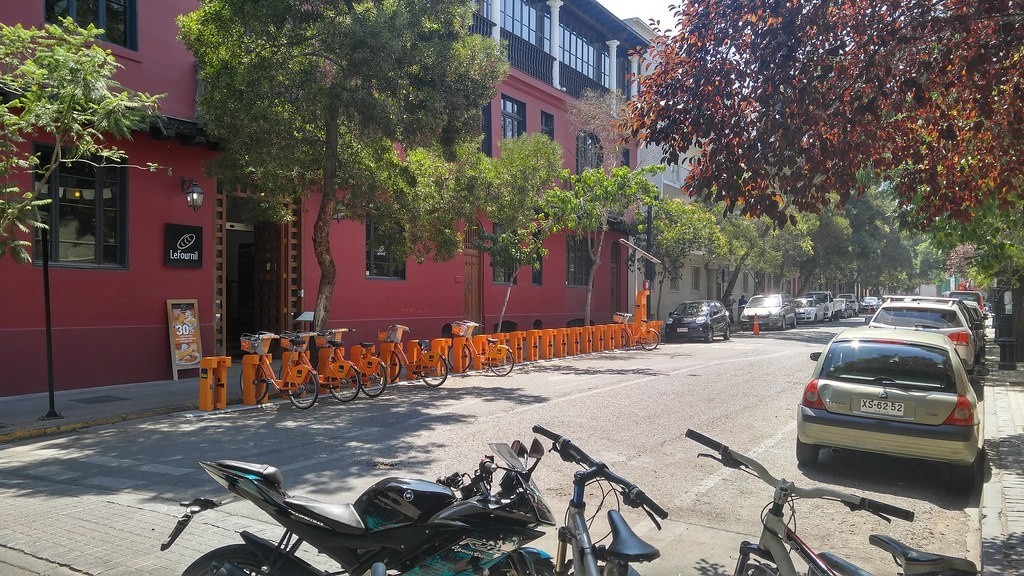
left=802, top=290, right=836, bottom=322
left=739, top=292, right=797, bottom=331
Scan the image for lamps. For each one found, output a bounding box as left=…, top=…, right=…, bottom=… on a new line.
left=332, top=213, right=344, bottom=223
left=183, top=176, right=205, bottom=212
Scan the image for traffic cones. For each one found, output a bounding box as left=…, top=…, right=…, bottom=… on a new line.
left=751, top=313, right=762, bottom=335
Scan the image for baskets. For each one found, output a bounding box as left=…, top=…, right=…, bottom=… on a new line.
left=613, top=314, right=629, bottom=323
left=452, top=324, right=474, bottom=338
left=240, top=336, right=272, bottom=355
left=280, top=334, right=310, bottom=352
left=314, top=331, right=343, bottom=347
left=378, top=327, right=403, bottom=343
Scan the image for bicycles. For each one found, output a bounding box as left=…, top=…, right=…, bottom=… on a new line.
left=373, top=323, right=449, bottom=388
left=239, top=330, right=319, bottom=409
left=612, top=312, right=660, bottom=351
left=304, top=327, right=388, bottom=398
left=531, top=422, right=670, bottom=576
left=685, top=428, right=979, bottom=576
left=448, top=320, right=515, bottom=376
left=278, top=328, right=362, bottom=403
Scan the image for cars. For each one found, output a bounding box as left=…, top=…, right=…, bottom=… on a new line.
left=664, top=299, right=732, bottom=344
left=868, top=287, right=995, bottom=385
left=791, top=297, right=826, bottom=325
left=796, top=325, right=990, bottom=493
left=832, top=293, right=884, bottom=320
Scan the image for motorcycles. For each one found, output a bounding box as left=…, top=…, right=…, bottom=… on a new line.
left=160, top=436, right=561, bottom=576
